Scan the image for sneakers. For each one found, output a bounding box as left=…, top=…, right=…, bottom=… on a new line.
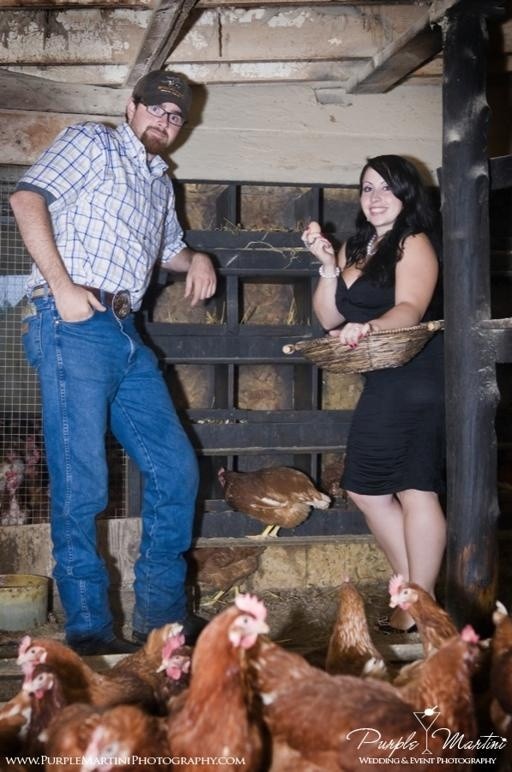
left=71, top=637, right=141, bottom=658
left=133, top=612, right=209, bottom=646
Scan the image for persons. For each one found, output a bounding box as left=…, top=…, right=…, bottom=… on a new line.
left=10, top=71, right=218, bottom=655
left=302, top=153, right=446, bottom=632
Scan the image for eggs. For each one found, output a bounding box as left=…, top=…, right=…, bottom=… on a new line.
left=308, top=221, right=321, bottom=233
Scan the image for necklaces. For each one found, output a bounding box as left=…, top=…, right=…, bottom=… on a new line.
left=367, top=234, right=381, bottom=257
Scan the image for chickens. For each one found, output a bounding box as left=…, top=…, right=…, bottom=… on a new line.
left=214, top=464, right=333, bottom=540
left=0, top=433, right=49, bottom=527
left=0, top=570, right=512, bottom=772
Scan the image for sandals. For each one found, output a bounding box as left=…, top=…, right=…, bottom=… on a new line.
left=373, top=611, right=416, bottom=636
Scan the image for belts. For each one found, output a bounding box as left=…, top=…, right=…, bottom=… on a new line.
left=32, top=283, right=131, bottom=320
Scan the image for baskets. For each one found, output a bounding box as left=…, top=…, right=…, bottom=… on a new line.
left=294, top=319, right=443, bottom=375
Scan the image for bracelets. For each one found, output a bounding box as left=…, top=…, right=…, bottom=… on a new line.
left=318, top=264, right=340, bottom=279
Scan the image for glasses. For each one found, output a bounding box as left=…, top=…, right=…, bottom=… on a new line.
left=145, top=104, right=185, bottom=128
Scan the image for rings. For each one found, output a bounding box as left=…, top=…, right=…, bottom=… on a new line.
left=307, top=241, right=313, bottom=246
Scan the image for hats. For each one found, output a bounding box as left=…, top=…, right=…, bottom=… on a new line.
left=133, top=70, right=193, bottom=114
